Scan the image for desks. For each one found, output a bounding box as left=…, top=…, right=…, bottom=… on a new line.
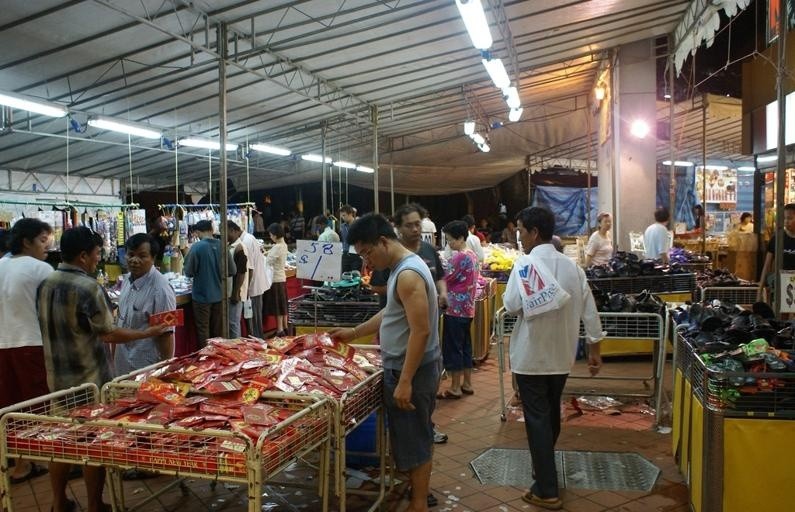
left=561, top=238, right=588, bottom=247
left=674, top=235, right=720, bottom=268
left=104, top=250, right=296, bottom=366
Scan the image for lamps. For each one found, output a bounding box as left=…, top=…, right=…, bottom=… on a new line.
left=87, top=113, right=163, bottom=139
left=301, top=153, right=374, bottom=173
left=179, top=135, right=238, bottom=151
left=0, top=89, right=69, bottom=118
left=456, top=0, right=493, bottom=49
left=464, top=57, right=523, bottom=152
left=249, top=142, right=292, bottom=155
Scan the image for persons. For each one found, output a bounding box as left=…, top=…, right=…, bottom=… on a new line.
left=380, top=201, right=518, bottom=265
left=737, top=212, right=753, bottom=232
left=437, top=221, right=481, bottom=401
left=148, top=206, right=288, bottom=349
left=585, top=210, right=614, bottom=267
left=325, top=212, right=444, bottom=499
left=291, top=208, right=357, bottom=287
left=35, top=226, right=170, bottom=511
left=756, top=204, right=795, bottom=321
left=693, top=205, right=707, bottom=231
left=500, top=206, right=608, bottom=511
left=368, top=203, right=448, bottom=447
left=642, top=205, right=674, bottom=268
left=114, top=233, right=176, bottom=478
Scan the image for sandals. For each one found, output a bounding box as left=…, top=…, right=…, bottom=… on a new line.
left=522, top=488, right=563, bottom=509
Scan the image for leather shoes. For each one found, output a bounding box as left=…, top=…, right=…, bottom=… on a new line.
left=580, top=247, right=794, bottom=410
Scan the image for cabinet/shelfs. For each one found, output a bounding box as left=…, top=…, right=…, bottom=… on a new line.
left=584, top=268, right=697, bottom=356
left=0, top=344, right=411, bottom=512
left=287, top=277, right=448, bottom=380
left=496, top=302, right=669, bottom=425
left=44, top=250, right=122, bottom=286
left=480, top=270, right=512, bottom=311
left=670, top=261, right=713, bottom=271
left=472, top=275, right=497, bottom=368
left=697, top=283, right=761, bottom=302
left=672, top=310, right=795, bottom=511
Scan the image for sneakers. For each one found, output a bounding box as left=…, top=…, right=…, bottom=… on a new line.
left=434, top=428, right=448, bottom=443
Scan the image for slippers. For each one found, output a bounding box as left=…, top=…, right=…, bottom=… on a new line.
left=435, top=386, right=475, bottom=399
left=294, top=270, right=380, bottom=323
left=9, top=462, right=49, bottom=484
